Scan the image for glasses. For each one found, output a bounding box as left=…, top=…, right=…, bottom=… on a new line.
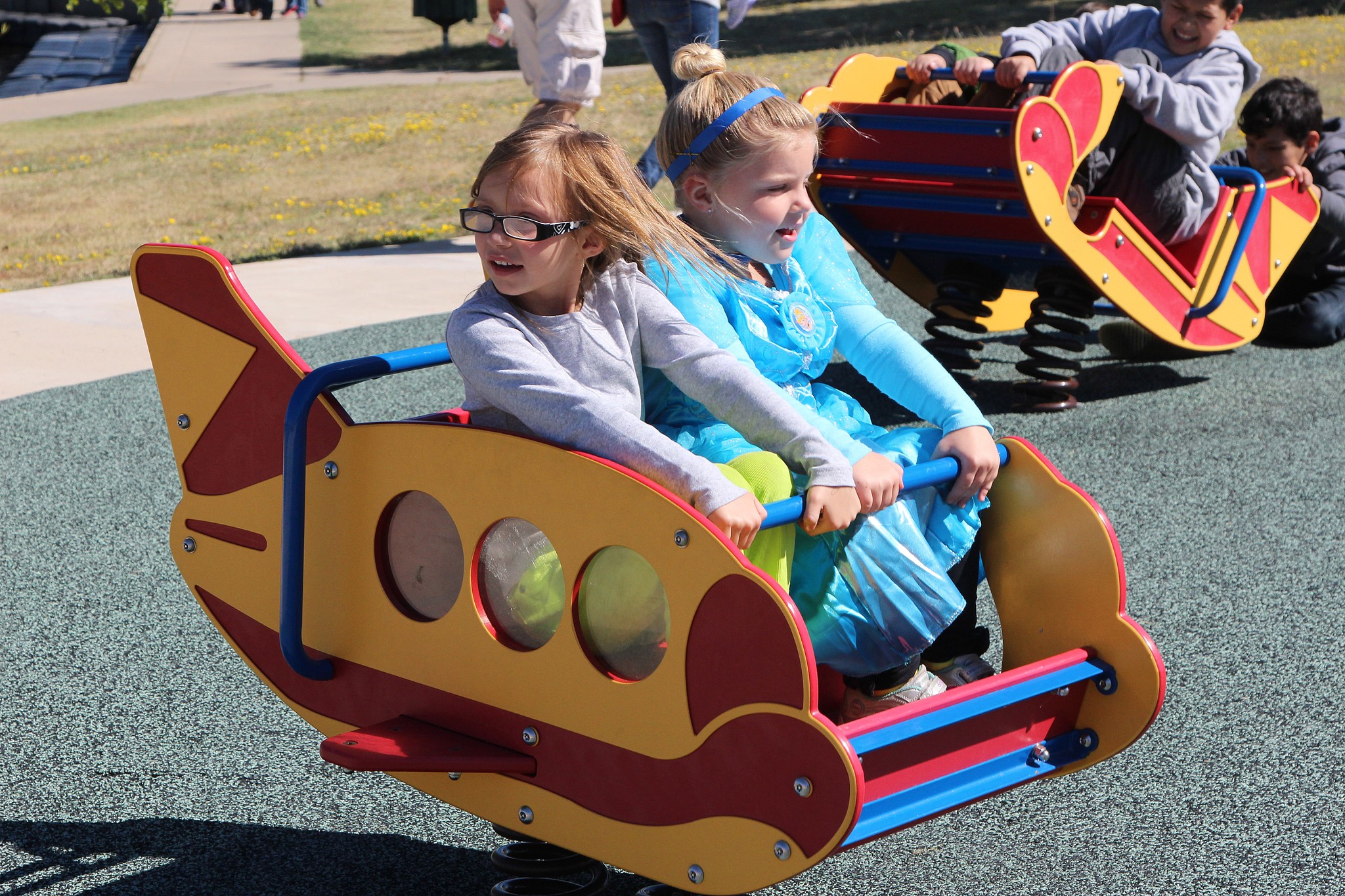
left=460, top=206, right=593, bottom=241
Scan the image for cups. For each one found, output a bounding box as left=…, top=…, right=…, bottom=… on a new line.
left=488, top=13, right=515, bottom=48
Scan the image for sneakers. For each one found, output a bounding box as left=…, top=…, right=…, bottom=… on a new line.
left=841, top=663, right=947, bottom=720
left=926, top=653, right=995, bottom=691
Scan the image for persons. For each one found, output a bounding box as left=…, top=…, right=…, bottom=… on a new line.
left=654, top=38, right=998, bottom=715
left=904, top=0, right=1263, bottom=248
left=445, top=116, right=862, bottom=598
left=610, top=0, right=757, bottom=191
left=484, top=0, right=606, bottom=125
left=211, top=0, right=326, bottom=20
left=1097, top=74, right=1345, bottom=363
